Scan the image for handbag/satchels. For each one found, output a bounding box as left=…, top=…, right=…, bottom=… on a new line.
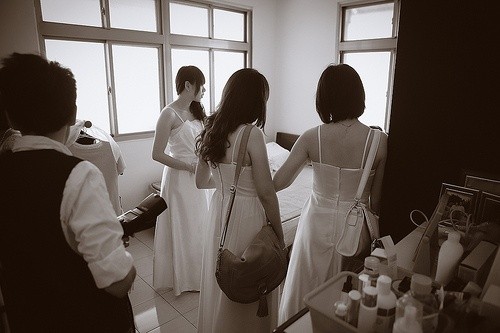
left=337, top=199, right=380, bottom=257
left=215, top=224, right=289, bottom=304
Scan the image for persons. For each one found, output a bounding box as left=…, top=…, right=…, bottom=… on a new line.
left=0, top=52, right=138, bottom=333
left=152, top=65, right=216, bottom=297
left=194, top=69, right=285, bottom=333
left=273, top=63, right=388, bottom=333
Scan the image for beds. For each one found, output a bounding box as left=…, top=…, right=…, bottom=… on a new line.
left=266, top=132, right=314, bottom=248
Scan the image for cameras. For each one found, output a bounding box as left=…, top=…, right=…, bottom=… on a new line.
left=116, top=193, right=167, bottom=247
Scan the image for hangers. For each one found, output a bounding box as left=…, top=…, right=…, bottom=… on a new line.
left=76, top=131, right=94, bottom=141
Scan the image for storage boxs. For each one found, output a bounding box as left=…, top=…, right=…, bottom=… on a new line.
left=458, top=240, right=497, bottom=283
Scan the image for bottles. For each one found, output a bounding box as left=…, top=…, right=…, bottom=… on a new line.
left=436, top=232, right=464, bottom=285
left=392, top=306, right=425, bottom=333
left=396, top=273, right=438, bottom=333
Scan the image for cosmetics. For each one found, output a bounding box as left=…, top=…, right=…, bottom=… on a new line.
left=435, top=231, right=464, bottom=287
left=334, top=257, right=439, bottom=333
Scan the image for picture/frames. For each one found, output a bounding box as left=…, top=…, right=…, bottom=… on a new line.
left=438, top=175, right=500, bottom=224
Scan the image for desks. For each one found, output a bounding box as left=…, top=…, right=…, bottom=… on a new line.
left=302, top=219, right=450, bottom=333
